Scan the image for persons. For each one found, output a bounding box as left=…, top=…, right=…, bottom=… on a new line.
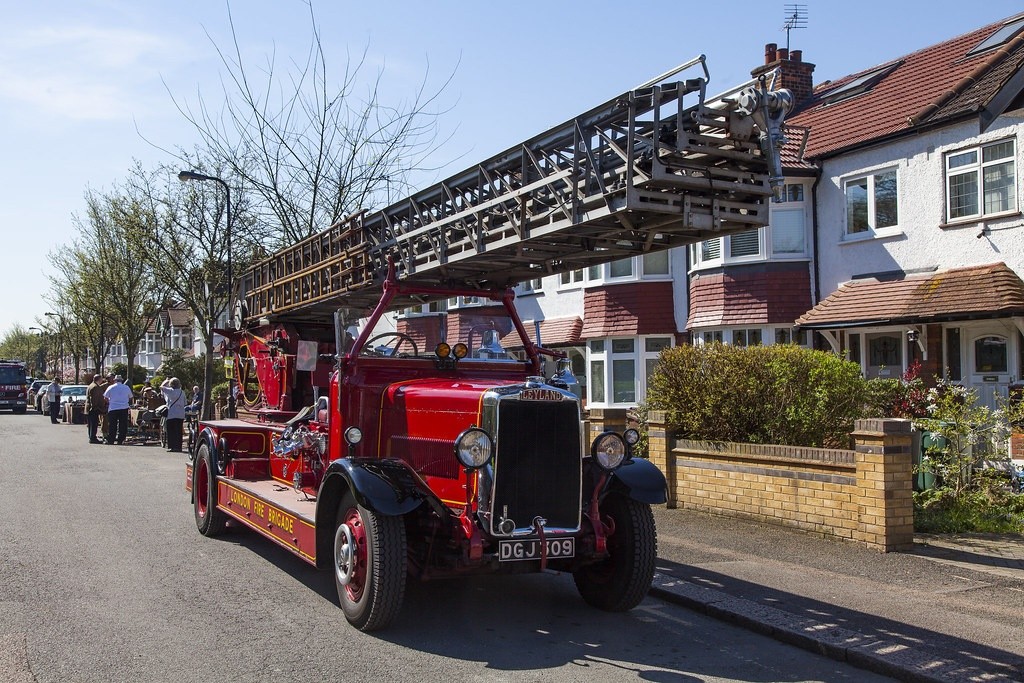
left=83, top=372, right=134, bottom=444
left=184, top=385, right=203, bottom=422
left=159, top=376, right=187, bottom=452
left=46, top=377, right=62, bottom=424
left=140, top=382, right=157, bottom=398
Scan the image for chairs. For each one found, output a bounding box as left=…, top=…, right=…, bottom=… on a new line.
left=311, top=352, right=337, bottom=424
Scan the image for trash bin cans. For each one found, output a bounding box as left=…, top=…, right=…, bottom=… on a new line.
left=915, top=417, right=953, bottom=492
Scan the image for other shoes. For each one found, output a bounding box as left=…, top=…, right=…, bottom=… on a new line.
left=167, top=448, right=177, bottom=452
left=56, top=420, right=60, bottom=423
left=177, top=449, right=182, bottom=452
left=89, top=439, right=103, bottom=444
left=52, top=421, right=56, bottom=424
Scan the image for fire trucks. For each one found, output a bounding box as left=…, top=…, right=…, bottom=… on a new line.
left=185, top=50, right=813, bottom=634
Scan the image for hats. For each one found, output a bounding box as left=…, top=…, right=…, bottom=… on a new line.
left=113, top=374, right=124, bottom=381
left=143, top=386, right=152, bottom=392
left=145, top=379, right=152, bottom=387
left=55, top=377, right=62, bottom=382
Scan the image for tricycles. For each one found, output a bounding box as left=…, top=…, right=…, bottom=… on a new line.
left=159, top=411, right=200, bottom=460
left=103, top=405, right=159, bottom=446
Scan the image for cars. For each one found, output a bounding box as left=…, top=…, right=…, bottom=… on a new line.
left=24, top=376, right=64, bottom=415
left=59, top=385, right=89, bottom=422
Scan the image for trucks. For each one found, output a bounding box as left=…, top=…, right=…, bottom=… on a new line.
left=0, top=359, right=34, bottom=415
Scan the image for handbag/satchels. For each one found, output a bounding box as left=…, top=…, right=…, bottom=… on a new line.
left=159, top=406, right=168, bottom=417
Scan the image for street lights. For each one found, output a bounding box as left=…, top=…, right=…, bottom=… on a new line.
left=178, top=169, right=235, bottom=421
left=29, top=327, right=42, bottom=337
left=381, top=174, right=390, bottom=207
left=45, top=313, right=63, bottom=385
